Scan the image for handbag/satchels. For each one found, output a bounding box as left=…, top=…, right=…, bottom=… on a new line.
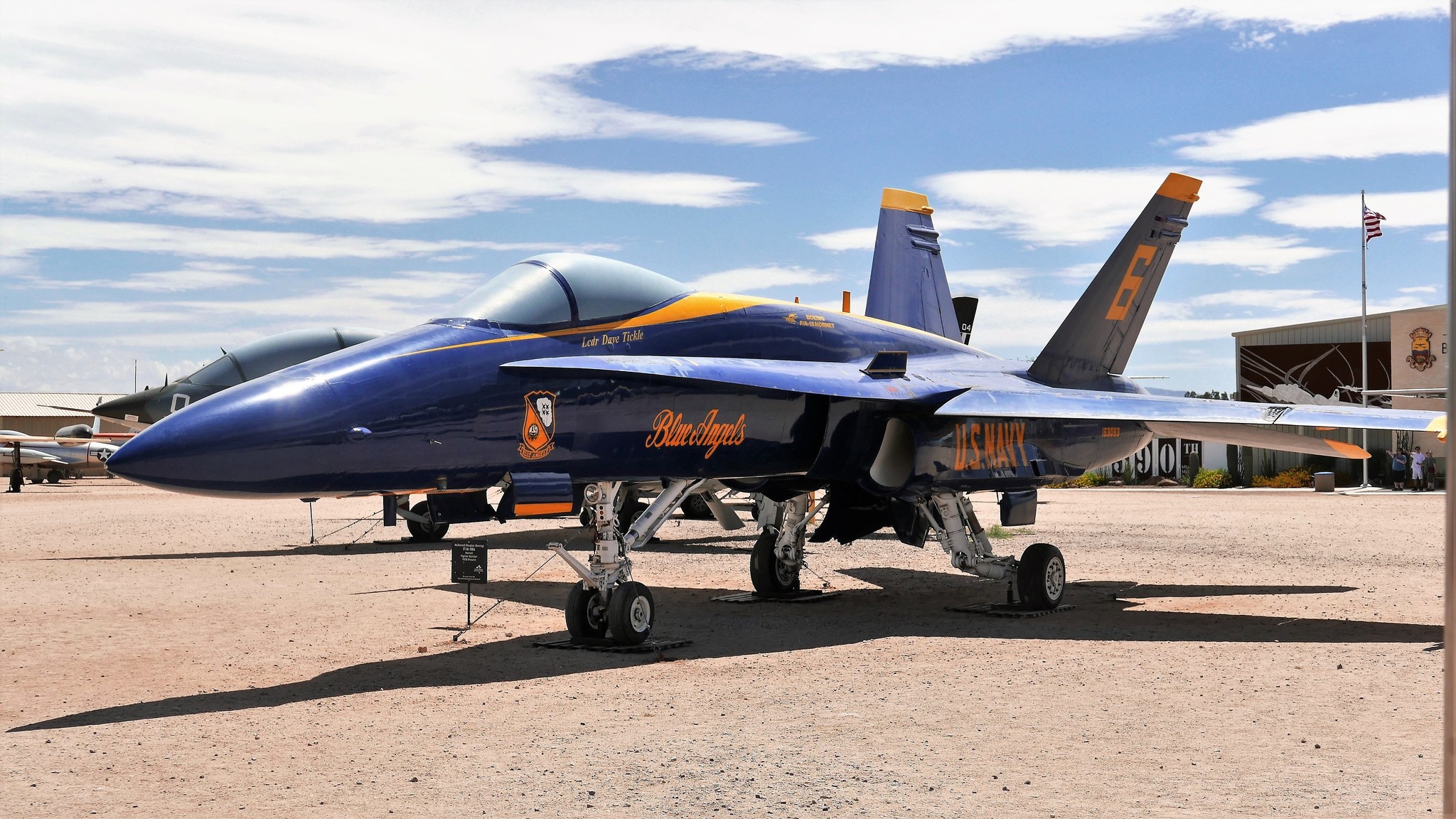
left=1427, top=467, right=1434, bottom=473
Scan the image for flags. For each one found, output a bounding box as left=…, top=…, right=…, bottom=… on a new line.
left=1363, top=206, right=1386, bottom=241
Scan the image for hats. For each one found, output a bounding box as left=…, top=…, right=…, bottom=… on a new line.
left=1426, top=449, right=1433, bottom=455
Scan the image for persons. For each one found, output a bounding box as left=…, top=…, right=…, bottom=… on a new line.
left=1386, top=448, right=1407, bottom=491
left=1416, top=450, right=1437, bottom=492
left=1403, top=446, right=1425, bottom=491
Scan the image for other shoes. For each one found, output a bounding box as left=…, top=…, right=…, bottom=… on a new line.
left=1425, top=488, right=1431, bottom=491
left=1398, top=488, right=1403, bottom=491
left=1419, top=489, right=1423, bottom=491
left=1412, top=489, right=1418, bottom=491
left=1431, top=489, right=1435, bottom=491
left=1393, top=488, right=1398, bottom=491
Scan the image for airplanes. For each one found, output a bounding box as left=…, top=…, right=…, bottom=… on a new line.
left=0, top=424, right=138, bottom=495
left=106, top=174, right=1449, bottom=650
left=91, top=328, right=711, bottom=542
